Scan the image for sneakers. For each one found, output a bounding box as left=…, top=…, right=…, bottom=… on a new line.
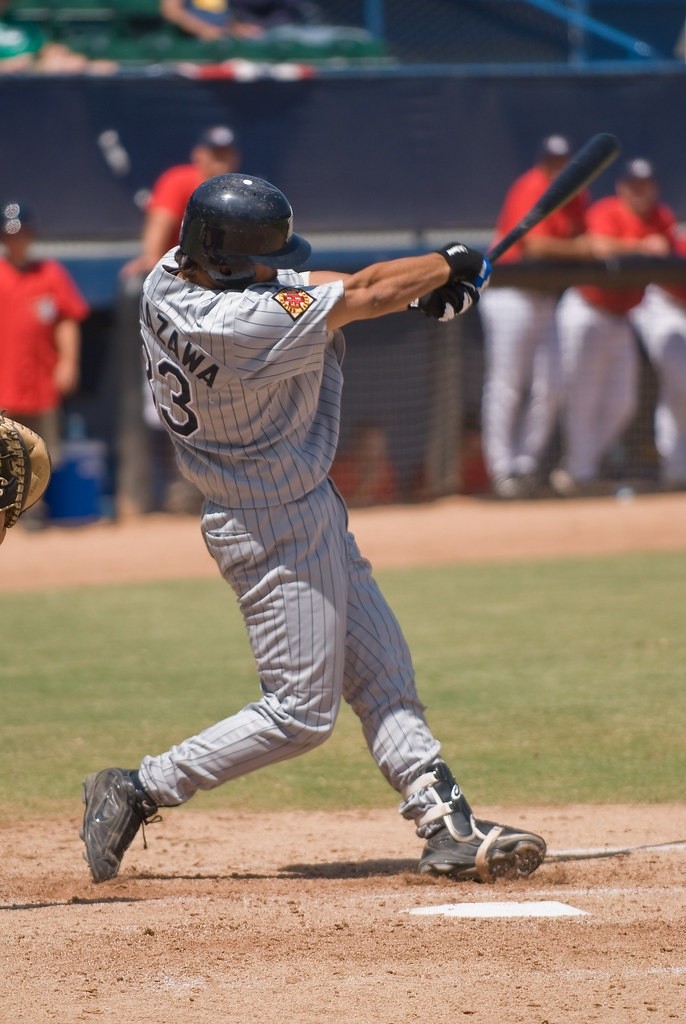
left=79, top=767, right=162, bottom=883
left=417, top=826, right=546, bottom=884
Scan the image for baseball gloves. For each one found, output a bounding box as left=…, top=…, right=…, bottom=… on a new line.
left=1, top=415, right=51, bottom=539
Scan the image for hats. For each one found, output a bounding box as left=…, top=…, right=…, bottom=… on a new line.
left=541, top=135, right=570, bottom=158
left=197, top=128, right=235, bottom=147
left=615, top=159, right=657, bottom=180
left=0, top=201, right=37, bottom=228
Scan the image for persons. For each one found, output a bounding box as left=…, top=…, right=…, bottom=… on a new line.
left=0, top=0, right=686, bottom=554
left=78, top=172, right=547, bottom=886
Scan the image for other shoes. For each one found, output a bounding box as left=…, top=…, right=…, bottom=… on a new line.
left=489, top=450, right=589, bottom=500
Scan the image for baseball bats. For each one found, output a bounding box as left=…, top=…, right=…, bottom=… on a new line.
left=487, top=132, right=621, bottom=270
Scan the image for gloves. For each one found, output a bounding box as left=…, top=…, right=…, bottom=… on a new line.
left=407, top=281, right=480, bottom=322
left=436, top=242, right=492, bottom=293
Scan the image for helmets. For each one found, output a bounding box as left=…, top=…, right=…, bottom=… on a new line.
left=179, top=173, right=312, bottom=291
